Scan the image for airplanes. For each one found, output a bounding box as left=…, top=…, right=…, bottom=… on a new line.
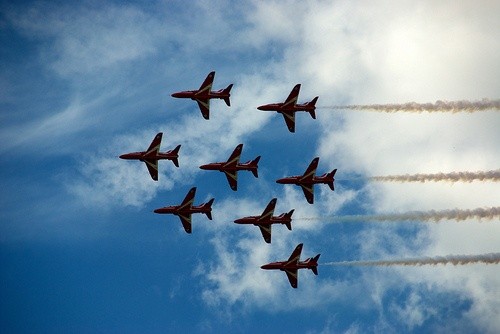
left=260, top=243, right=320, bottom=288
left=199, top=144, right=261, bottom=191
left=119, top=132, right=180, bottom=182
left=276, top=157, right=336, bottom=204
left=151, top=187, right=215, bottom=234
left=257, top=83, right=318, bottom=133
left=234, top=198, right=294, bottom=244
left=171, top=71, right=234, bottom=122
